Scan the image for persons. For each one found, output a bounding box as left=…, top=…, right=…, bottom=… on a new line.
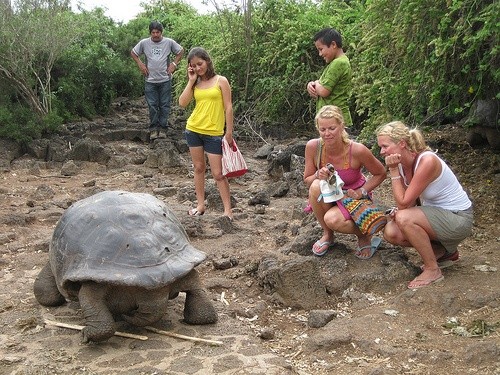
left=130, top=21, right=184, bottom=140
left=375, top=121, right=473, bottom=290
left=303, top=104, right=387, bottom=261
left=179, top=47, right=233, bottom=222
left=306, top=28, right=352, bottom=139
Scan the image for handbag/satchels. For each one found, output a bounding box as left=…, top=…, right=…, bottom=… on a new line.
left=221, top=137, right=248, bottom=177
left=341, top=190, right=388, bottom=237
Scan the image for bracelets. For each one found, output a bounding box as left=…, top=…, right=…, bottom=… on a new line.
left=390, top=176, right=401, bottom=180
left=172, top=62, right=178, bottom=68
left=315, top=170, right=320, bottom=178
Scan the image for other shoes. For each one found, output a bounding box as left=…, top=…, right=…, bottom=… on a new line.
left=304, top=203, right=313, bottom=213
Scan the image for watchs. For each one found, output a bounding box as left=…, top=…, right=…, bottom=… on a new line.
left=360, top=187, right=367, bottom=197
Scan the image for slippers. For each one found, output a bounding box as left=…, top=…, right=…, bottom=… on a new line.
left=355, top=237, right=381, bottom=260
left=418, top=251, right=459, bottom=270
left=408, top=274, right=444, bottom=289
left=188, top=209, right=204, bottom=216
left=222, top=215, right=233, bottom=221
left=312, top=238, right=337, bottom=255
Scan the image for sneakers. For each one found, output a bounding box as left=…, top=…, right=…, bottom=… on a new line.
left=159, top=130, right=166, bottom=138
left=150, top=130, right=158, bottom=140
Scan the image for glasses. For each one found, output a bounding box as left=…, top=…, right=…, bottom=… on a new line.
left=325, top=163, right=336, bottom=185
